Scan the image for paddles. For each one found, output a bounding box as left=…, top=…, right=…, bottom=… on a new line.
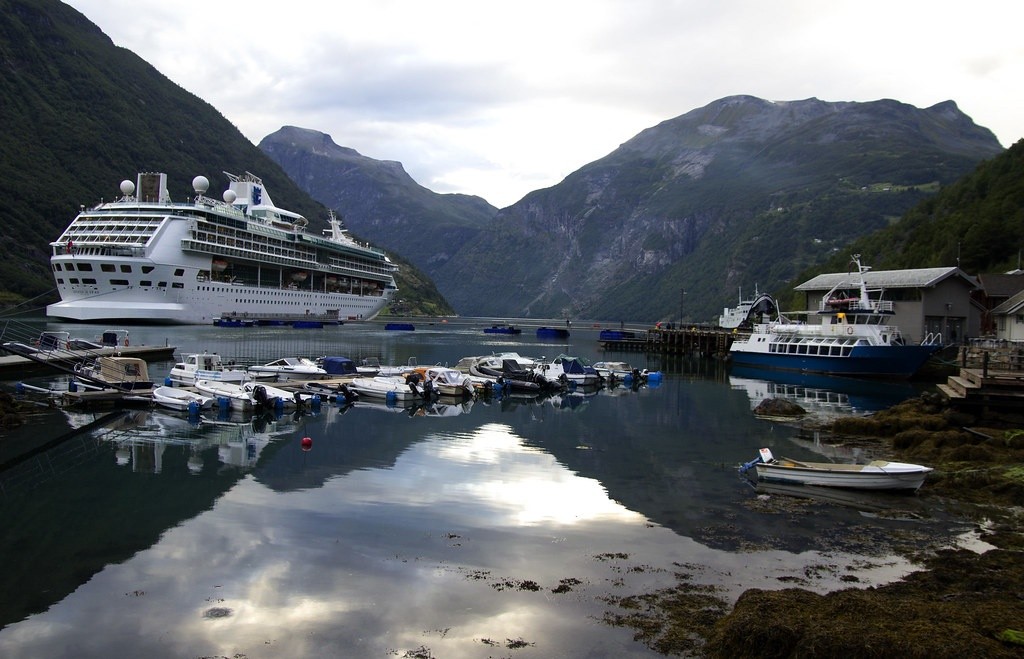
left=781, top=455, right=816, bottom=469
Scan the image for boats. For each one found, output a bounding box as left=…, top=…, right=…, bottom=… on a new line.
left=242, top=381, right=317, bottom=414
left=722, top=256, right=961, bottom=380
left=536, top=327, right=571, bottom=338
left=718, top=283, right=778, bottom=329
left=45, top=169, right=401, bottom=327
left=484, top=326, right=521, bottom=335
left=737, top=449, right=934, bottom=493
left=195, top=379, right=272, bottom=414
left=599, top=329, right=637, bottom=341
left=67, top=350, right=634, bottom=417
left=292, top=321, right=323, bottom=329
left=384, top=324, right=415, bottom=331
left=593, top=361, right=662, bottom=384
left=151, top=383, right=215, bottom=413
left=218, top=317, right=251, bottom=327
left=754, top=481, right=928, bottom=519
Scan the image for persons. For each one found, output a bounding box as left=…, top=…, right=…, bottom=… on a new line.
left=666, top=322, right=671, bottom=331
left=569, top=320, right=572, bottom=327
left=951, top=327, right=956, bottom=343
left=204, top=350, right=208, bottom=354
left=566, top=318, right=569, bottom=326
left=621, top=319, right=624, bottom=329
left=672, top=322, right=676, bottom=329
left=656, top=320, right=661, bottom=329
left=964, top=330, right=969, bottom=346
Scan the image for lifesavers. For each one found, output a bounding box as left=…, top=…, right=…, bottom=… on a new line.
left=125, top=338, right=130, bottom=346
left=35, top=340, right=40, bottom=347
left=847, top=327, right=854, bottom=335
